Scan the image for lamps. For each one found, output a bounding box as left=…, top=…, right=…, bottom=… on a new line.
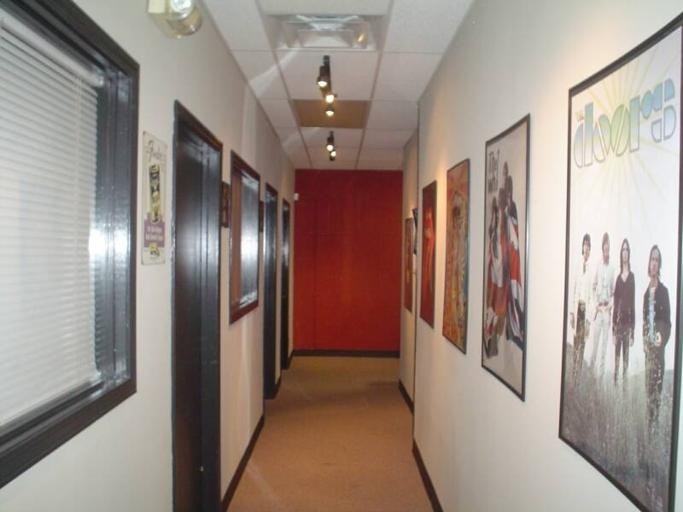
left=309, top=52, right=345, bottom=165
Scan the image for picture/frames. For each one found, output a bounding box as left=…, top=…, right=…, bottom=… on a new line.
left=226, top=149, right=262, bottom=327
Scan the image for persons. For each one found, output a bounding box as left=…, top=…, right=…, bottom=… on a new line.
left=591, top=230, right=619, bottom=393
left=569, top=231, right=595, bottom=403
left=643, top=245, right=669, bottom=434
left=611, top=239, right=636, bottom=388
left=485, top=147, right=522, bottom=362
